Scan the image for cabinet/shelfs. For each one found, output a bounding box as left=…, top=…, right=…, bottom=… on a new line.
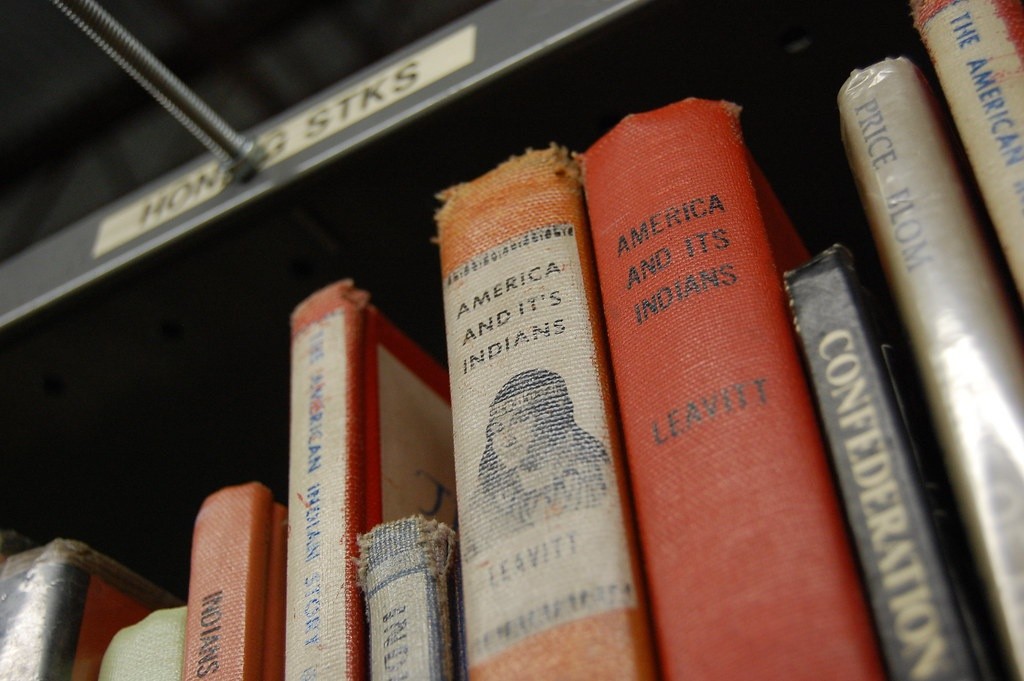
left=2, top=0, right=1023, bottom=681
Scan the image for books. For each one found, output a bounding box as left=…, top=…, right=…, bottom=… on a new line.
left=0, top=535, right=186, bottom=679
left=832, top=58, right=1024, bottom=680
left=182, top=478, right=289, bottom=681
left=428, top=143, right=661, bottom=681
left=785, top=245, right=1002, bottom=681
left=287, top=276, right=453, bottom=681
left=570, top=98, right=885, bottom=681
left=355, top=514, right=468, bottom=681
left=97, top=605, right=188, bottom=679
left=917, top=0, right=1024, bottom=300
